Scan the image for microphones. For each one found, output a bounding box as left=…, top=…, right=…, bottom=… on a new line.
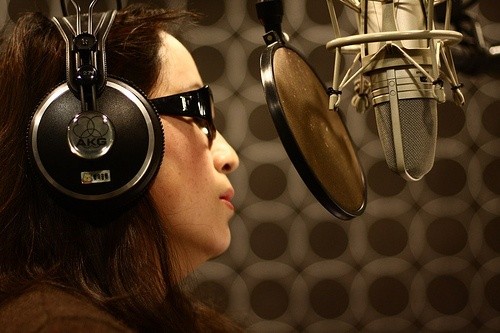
left=353, top=0, right=439, bottom=184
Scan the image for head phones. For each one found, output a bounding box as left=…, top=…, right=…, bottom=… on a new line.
left=24, top=1, right=168, bottom=225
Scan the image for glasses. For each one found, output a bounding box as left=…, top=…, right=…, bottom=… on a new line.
left=151, top=84, right=218, bottom=139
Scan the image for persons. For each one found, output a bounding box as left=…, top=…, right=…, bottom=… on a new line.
left=0, top=13, right=240, bottom=333
